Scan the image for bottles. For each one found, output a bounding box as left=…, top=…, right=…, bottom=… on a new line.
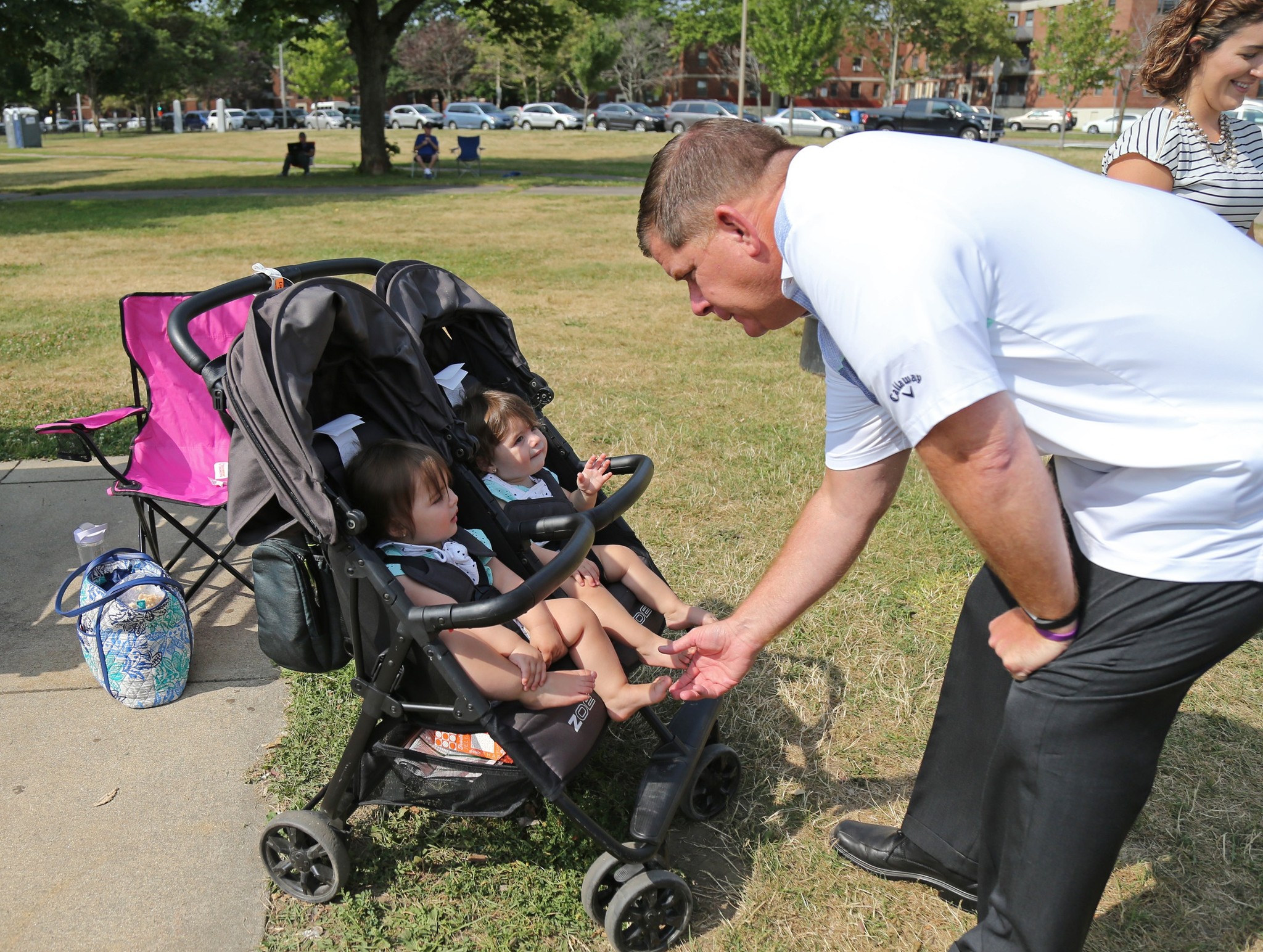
left=73, top=521, right=108, bottom=566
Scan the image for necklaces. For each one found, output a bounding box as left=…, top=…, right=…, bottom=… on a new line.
left=1172, top=93, right=1238, bottom=170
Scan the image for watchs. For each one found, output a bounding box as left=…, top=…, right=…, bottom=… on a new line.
left=1018, top=578, right=1082, bottom=632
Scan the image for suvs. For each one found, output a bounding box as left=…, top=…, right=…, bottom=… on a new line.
left=1007, top=108, right=1077, bottom=134
left=663, top=99, right=760, bottom=134
left=343, top=104, right=391, bottom=130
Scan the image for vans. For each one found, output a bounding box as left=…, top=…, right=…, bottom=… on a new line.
left=311, top=101, right=351, bottom=115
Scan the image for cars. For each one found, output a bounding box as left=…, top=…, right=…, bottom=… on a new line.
left=388, top=103, right=446, bottom=131
left=0, top=112, right=186, bottom=136
left=502, top=101, right=584, bottom=131
left=303, top=108, right=344, bottom=130
left=1082, top=112, right=1143, bottom=135
left=441, top=101, right=515, bottom=131
left=591, top=101, right=670, bottom=133
left=207, top=108, right=247, bottom=132
left=181, top=110, right=210, bottom=133
left=760, top=107, right=865, bottom=139
left=271, top=107, right=310, bottom=130
left=241, top=107, right=275, bottom=130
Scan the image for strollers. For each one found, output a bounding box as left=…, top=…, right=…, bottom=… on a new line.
left=165, top=259, right=744, bottom=952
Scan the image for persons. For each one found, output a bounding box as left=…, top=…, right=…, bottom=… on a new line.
left=281, top=132, right=315, bottom=176
left=635, top=117, right=1263, bottom=952
left=454, top=390, right=719, bottom=669
left=413, top=122, right=439, bottom=180
left=341, top=436, right=671, bottom=722
left=1065, top=109, right=1074, bottom=130
left=1100, top=0, right=1263, bottom=243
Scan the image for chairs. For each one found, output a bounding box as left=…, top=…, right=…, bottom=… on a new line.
left=288, top=141, right=316, bottom=172
left=33, top=292, right=257, bottom=604
left=412, top=150, right=439, bottom=179
left=449, top=135, right=486, bottom=177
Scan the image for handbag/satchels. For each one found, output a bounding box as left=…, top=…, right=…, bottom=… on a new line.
left=56, top=548, right=193, bottom=709
left=253, top=535, right=352, bottom=673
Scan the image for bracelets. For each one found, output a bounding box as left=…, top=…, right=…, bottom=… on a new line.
left=1030, top=625, right=1080, bottom=642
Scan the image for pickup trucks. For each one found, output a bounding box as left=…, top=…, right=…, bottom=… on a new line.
left=862, top=97, right=1006, bottom=144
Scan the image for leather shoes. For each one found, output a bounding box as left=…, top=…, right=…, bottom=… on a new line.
left=829, top=820, right=978, bottom=914
